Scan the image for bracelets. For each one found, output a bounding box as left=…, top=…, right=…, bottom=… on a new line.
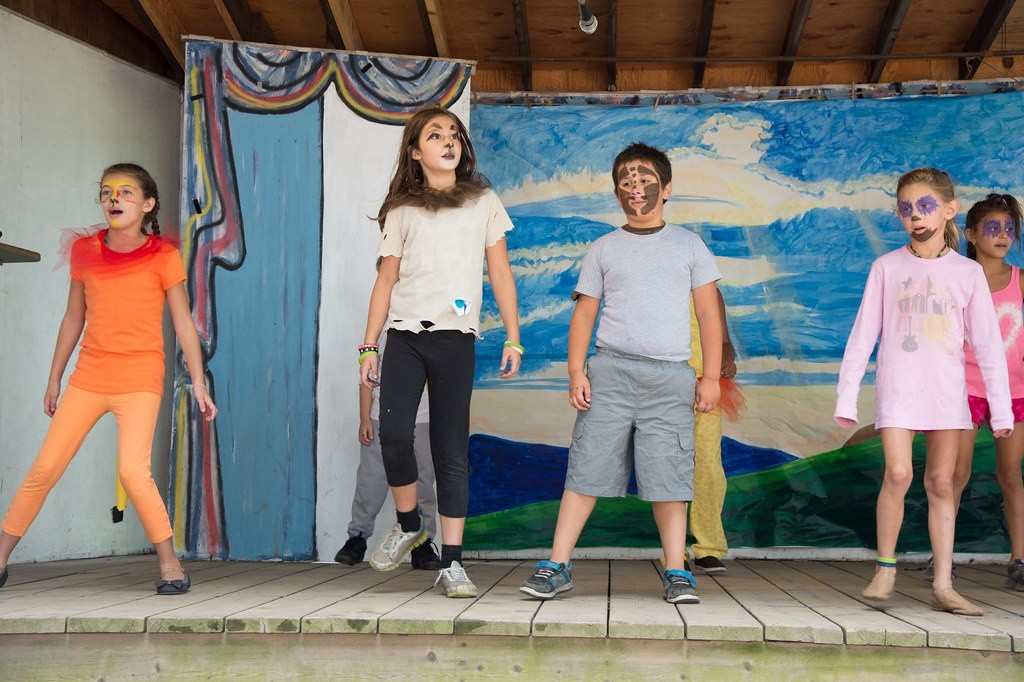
left=357, top=344, right=379, bottom=364
left=504, top=340, right=525, bottom=356
left=190, top=383, right=208, bottom=390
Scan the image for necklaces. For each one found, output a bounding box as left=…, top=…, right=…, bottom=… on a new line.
left=910, top=242, right=947, bottom=259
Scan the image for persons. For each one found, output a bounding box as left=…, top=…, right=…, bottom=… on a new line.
left=833, top=167, right=1014, bottom=616
left=335, top=108, right=525, bottom=598
left=660, top=237, right=737, bottom=576
left=922, top=194, right=1024, bottom=590
left=0, top=163, right=219, bottom=594
left=519, top=141, right=723, bottom=603
left=334, top=256, right=443, bottom=570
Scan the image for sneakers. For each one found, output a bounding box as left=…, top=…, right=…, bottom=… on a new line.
left=411, top=538, right=440, bottom=570
left=1005, top=559, right=1024, bottom=591
left=923, top=557, right=956, bottom=582
left=662, top=569, right=700, bottom=604
left=334, top=532, right=367, bottom=566
left=519, top=560, right=572, bottom=600
left=370, top=515, right=429, bottom=571
left=434, top=560, right=477, bottom=598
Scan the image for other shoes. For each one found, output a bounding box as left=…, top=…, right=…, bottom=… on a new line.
left=863, top=556, right=896, bottom=601
left=155, top=568, right=189, bottom=594
left=694, top=556, right=727, bottom=572
left=932, top=589, right=984, bottom=616
